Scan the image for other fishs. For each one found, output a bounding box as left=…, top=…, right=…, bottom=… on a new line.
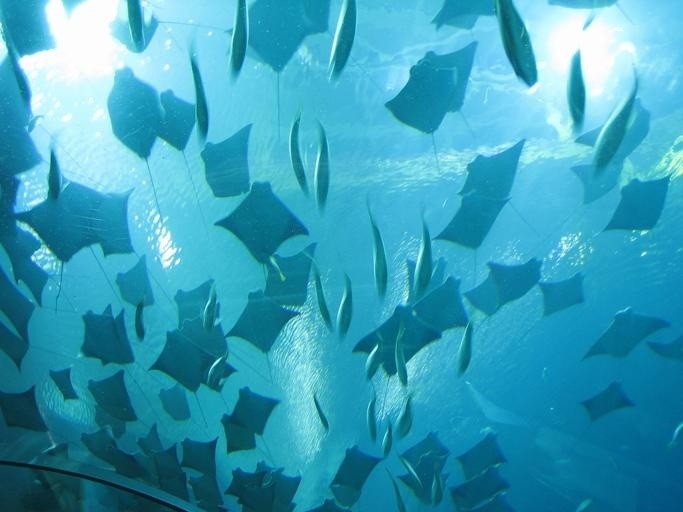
left=0, top=1, right=683, bottom=512
